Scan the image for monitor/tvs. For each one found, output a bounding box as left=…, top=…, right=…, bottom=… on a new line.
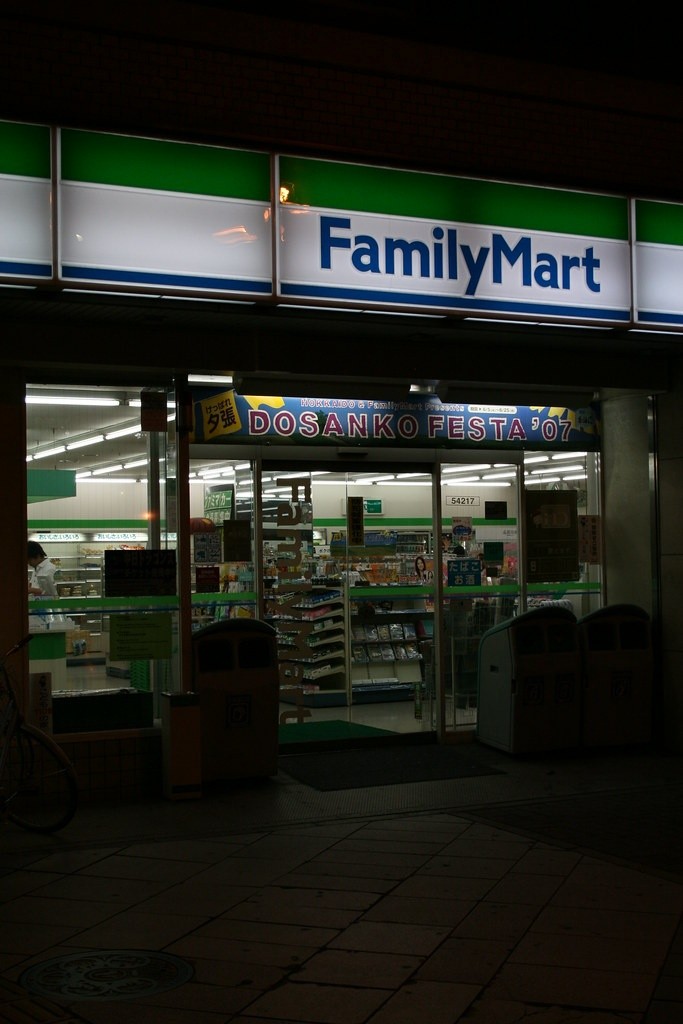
left=36, top=574, right=57, bottom=596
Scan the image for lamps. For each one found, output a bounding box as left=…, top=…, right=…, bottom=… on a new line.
left=408, top=383, right=435, bottom=393
left=184, top=373, right=239, bottom=389
left=25, top=396, right=588, bottom=486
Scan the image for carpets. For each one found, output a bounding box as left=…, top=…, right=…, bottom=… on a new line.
left=277, top=718, right=402, bottom=746
left=278, top=742, right=509, bottom=792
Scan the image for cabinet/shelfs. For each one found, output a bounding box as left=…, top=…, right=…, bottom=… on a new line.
left=279, top=595, right=346, bottom=693
left=351, top=636, right=423, bottom=663
left=53, top=568, right=101, bottom=596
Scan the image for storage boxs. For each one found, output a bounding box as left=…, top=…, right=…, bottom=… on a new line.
left=350, top=622, right=423, bottom=685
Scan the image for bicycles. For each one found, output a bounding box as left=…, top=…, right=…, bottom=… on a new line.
left=1, top=633, right=80, bottom=837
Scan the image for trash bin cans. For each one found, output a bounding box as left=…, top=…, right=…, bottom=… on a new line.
left=193, top=617, right=282, bottom=783
left=582, top=604, right=655, bottom=750
left=475, top=608, right=582, bottom=755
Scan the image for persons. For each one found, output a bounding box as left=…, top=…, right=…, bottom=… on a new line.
left=27, top=541, right=60, bottom=597
left=415, top=557, right=433, bottom=583
left=443, top=533, right=466, bottom=557
